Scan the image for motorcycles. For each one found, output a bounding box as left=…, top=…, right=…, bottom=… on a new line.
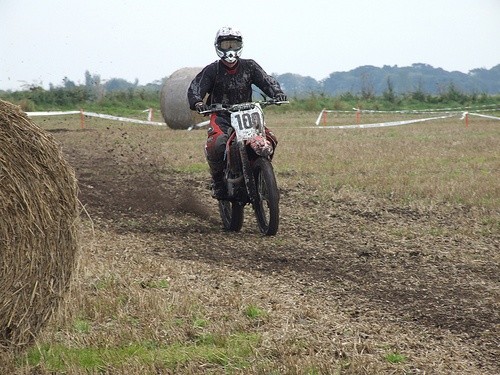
left=194, top=97, right=290, bottom=235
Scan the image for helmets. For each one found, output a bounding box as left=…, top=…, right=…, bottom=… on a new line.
left=214, top=26, right=243, bottom=64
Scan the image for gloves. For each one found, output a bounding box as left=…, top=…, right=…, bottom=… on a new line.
left=194, top=102, right=213, bottom=116
left=274, top=92, right=288, bottom=106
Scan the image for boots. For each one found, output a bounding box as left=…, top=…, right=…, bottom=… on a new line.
left=207, top=155, right=229, bottom=195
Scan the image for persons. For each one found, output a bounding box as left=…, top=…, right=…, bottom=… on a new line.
left=188, top=24, right=288, bottom=199
